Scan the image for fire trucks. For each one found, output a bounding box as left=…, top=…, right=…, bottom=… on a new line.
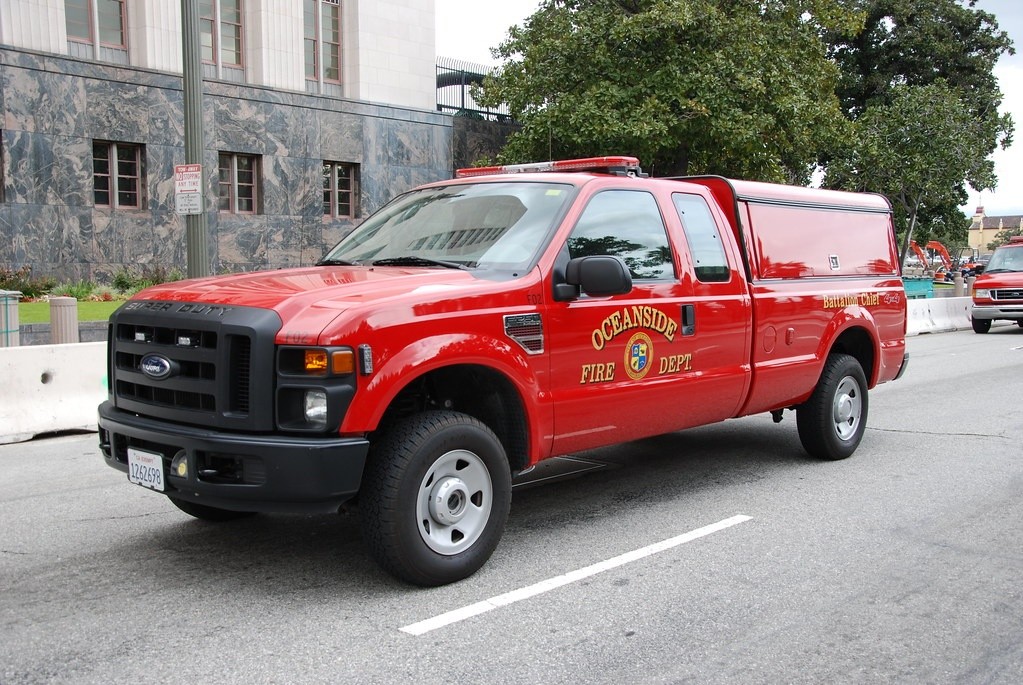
left=971, top=236, right=1023, bottom=334
left=97, top=156, right=909, bottom=588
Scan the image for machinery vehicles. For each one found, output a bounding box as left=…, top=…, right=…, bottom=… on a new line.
left=910, top=240, right=989, bottom=284
